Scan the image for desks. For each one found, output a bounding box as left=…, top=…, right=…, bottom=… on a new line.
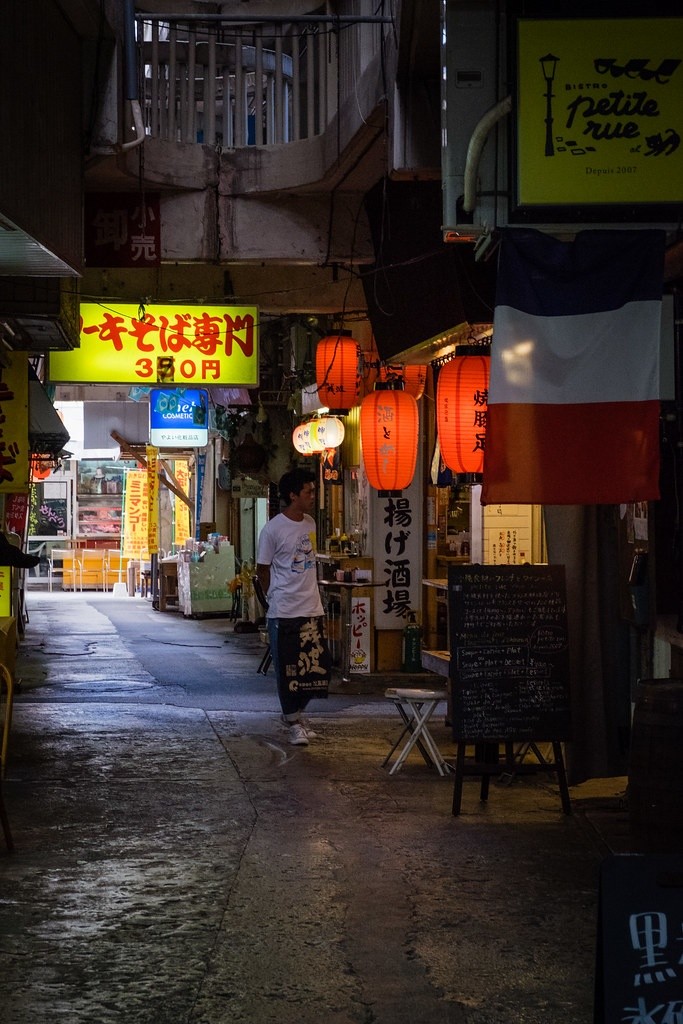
left=318, top=580, right=387, bottom=695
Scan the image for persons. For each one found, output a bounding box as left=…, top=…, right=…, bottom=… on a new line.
left=255, top=469, right=330, bottom=743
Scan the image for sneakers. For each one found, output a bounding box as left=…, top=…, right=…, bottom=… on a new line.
left=288, top=724, right=317, bottom=745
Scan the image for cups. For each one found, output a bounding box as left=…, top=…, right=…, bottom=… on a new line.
left=334, top=570, right=344, bottom=581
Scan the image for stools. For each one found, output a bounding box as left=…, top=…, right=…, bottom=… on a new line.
left=382, top=688, right=451, bottom=777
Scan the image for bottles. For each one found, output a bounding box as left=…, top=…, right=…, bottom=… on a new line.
left=402, top=612, right=426, bottom=670
left=343, top=564, right=352, bottom=582
left=330, top=533, right=339, bottom=551
left=341, top=533, right=349, bottom=552
left=325, top=535, right=331, bottom=551
left=461, top=538, right=470, bottom=555
left=446, top=540, right=457, bottom=556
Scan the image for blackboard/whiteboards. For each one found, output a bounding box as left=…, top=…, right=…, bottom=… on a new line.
left=446, top=562, right=574, bottom=741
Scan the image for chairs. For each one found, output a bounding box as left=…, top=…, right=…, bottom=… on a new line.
left=106, top=549, right=128, bottom=592
left=47, top=549, right=77, bottom=592
left=78, top=549, right=105, bottom=593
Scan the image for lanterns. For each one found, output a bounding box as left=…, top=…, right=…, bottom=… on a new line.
left=361, top=381, right=419, bottom=499
left=436, top=345, right=491, bottom=487
left=315, top=329, right=363, bottom=416
left=292, top=349, right=427, bottom=456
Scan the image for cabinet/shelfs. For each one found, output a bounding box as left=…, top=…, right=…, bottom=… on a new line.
left=77, top=493, right=124, bottom=538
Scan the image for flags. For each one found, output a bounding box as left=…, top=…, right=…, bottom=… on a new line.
left=479, top=225, right=663, bottom=505
